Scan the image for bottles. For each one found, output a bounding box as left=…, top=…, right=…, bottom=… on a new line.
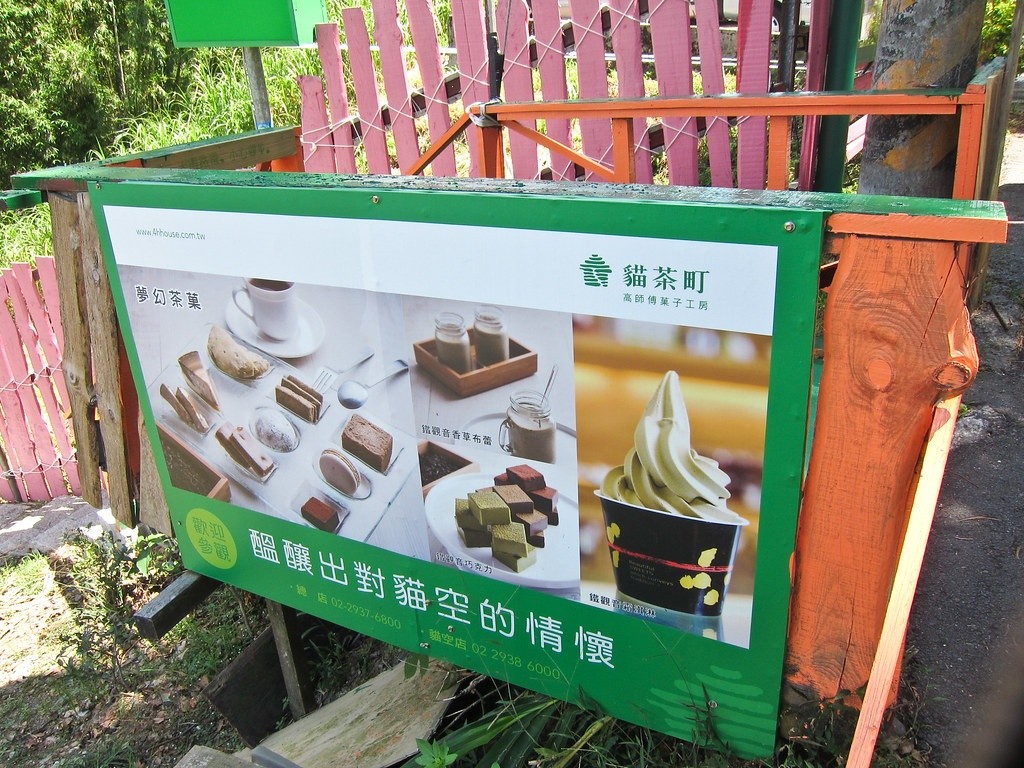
left=473, top=305, right=509, bottom=369
left=499, top=391, right=556, bottom=464
left=434, top=312, right=472, bottom=375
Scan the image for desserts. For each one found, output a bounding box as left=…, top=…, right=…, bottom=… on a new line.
left=453, top=465, right=560, bottom=573
left=158, top=351, right=394, bottom=532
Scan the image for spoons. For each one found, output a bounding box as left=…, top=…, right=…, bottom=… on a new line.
left=337, top=359, right=408, bottom=409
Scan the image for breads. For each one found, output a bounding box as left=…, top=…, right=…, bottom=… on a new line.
left=208, top=326, right=271, bottom=378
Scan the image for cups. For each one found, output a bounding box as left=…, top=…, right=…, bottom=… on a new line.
left=232, top=275, right=298, bottom=341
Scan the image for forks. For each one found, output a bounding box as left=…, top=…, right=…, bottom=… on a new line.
left=310, top=351, right=374, bottom=394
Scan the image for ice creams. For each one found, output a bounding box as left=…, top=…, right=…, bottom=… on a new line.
left=593, top=370, right=748, bottom=617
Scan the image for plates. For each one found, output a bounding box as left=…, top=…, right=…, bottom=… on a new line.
left=226, top=296, right=325, bottom=358
left=425, top=474, right=580, bottom=589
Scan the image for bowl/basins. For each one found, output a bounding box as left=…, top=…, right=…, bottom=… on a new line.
left=595, top=490, right=748, bottom=616
